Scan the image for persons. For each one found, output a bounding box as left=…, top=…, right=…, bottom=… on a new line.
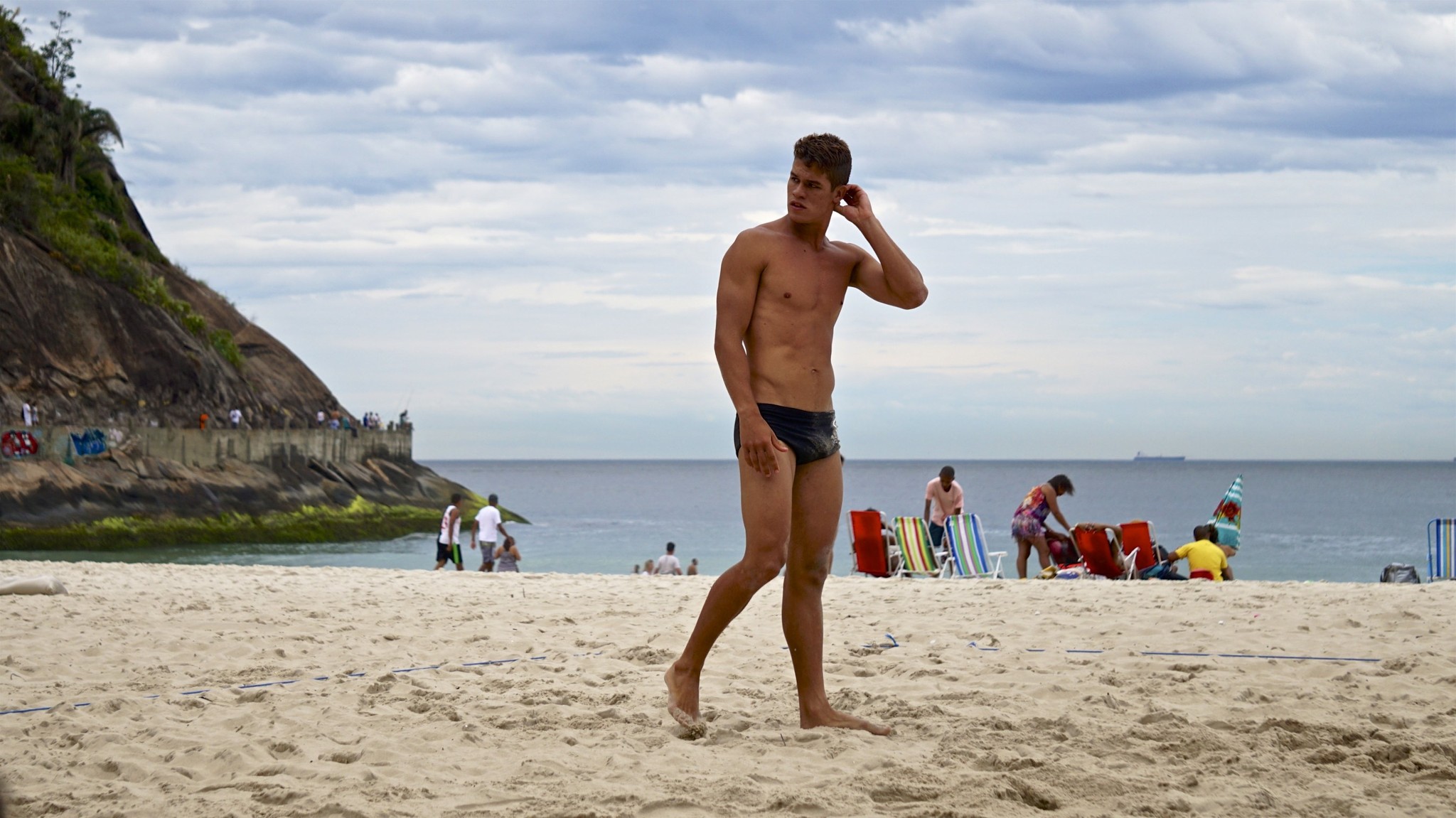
left=433, top=493, right=697, bottom=575
left=853, top=465, right=1239, bottom=582
left=663, top=132, right=928, bottom=737
left=22, top=398, right=412, bottom=432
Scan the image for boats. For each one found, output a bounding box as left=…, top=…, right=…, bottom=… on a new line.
left=1134, top=452, right=1184, bottom=462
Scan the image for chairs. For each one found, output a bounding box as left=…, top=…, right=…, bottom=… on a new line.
left=891, top=517, right=948, bottom=578
left=1069, top=527, right=1141, bottom=579
left=1116, top=520, right=1168, bottom=566
left=846, top=511, right=900, bottom=577
left=940, top=513, right=1008, bottom=579
left=1426, top=518, right=1456, bottom=583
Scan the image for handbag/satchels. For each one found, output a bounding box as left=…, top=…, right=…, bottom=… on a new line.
left=1033, top=563, right=1086, bottom=579
left=1380, top=562, right=1421, bottom=584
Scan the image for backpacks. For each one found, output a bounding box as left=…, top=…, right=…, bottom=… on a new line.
left=1140, top=560, right=1171, bottom=580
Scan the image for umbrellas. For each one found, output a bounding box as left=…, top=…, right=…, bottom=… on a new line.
left=1206, top=473, right=1246, bottom=549
